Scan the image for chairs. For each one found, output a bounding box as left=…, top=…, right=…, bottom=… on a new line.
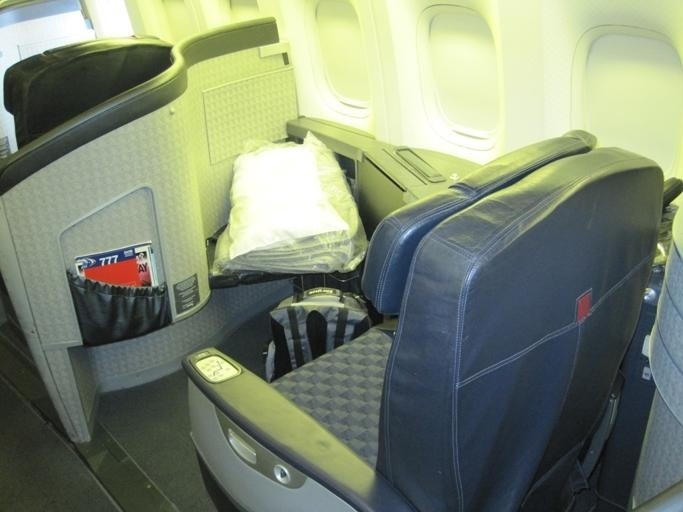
left=2, top=35, right=172, bottom=145
left=182, top=131, right=667, bottom=511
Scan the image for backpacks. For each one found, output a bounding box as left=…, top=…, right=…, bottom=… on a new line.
left=264, top=287, right=373, bottom=384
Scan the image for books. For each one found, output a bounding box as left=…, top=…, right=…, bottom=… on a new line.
left=74, top=241, right=160, bottom=286
